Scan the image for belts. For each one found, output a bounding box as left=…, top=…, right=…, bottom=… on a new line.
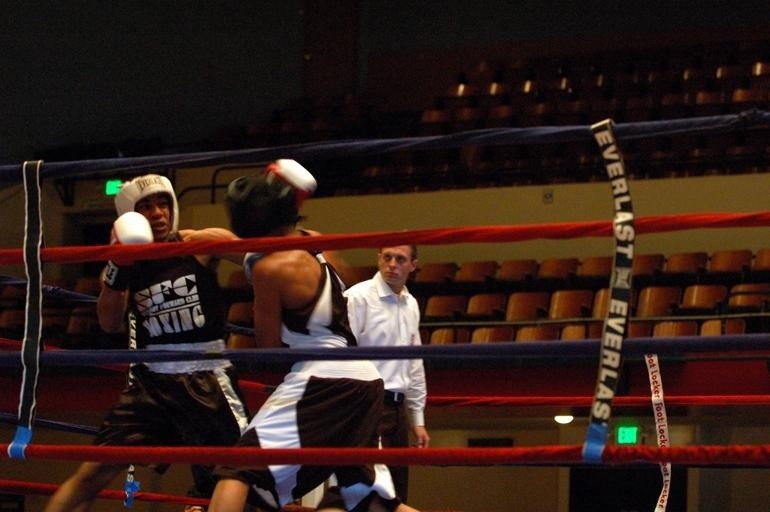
left=384, top=390, right=405, bottom=402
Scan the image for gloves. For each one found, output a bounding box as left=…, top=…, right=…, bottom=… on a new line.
left=101, top=212, right=154, bottom=290
left=267, top=159, right=317, bottom=211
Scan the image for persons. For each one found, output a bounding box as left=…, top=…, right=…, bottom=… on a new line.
left=177, top=176, right=424, bottom=512
left=42, top=158, right=318, bottom=512
left=341, top=245, right=430, bottom=501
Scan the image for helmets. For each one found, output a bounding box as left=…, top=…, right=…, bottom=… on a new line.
left=226, top=174, right=296, bottom=239
left=113, top=172, right=180, bottom=240
